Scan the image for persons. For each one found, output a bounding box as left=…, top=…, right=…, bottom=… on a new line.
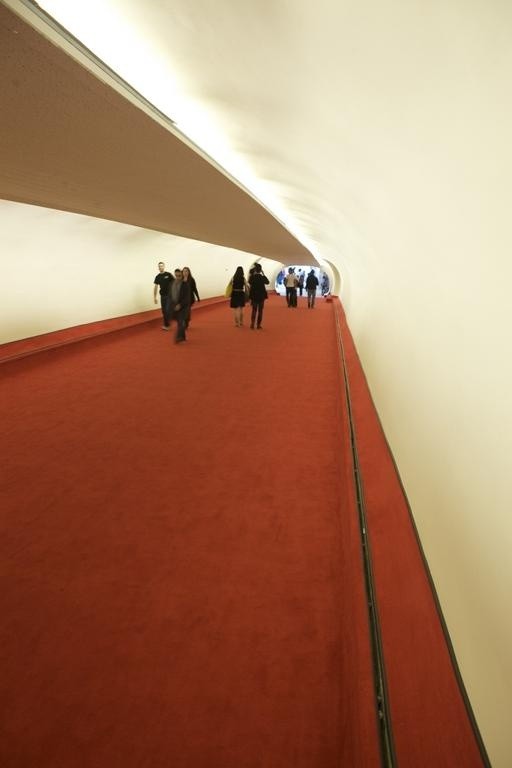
left=229, top=263, right=270, bottom=330
left=275, top=265, right=329, bottom=308
left=153, top=261, right=202, bottom=343
